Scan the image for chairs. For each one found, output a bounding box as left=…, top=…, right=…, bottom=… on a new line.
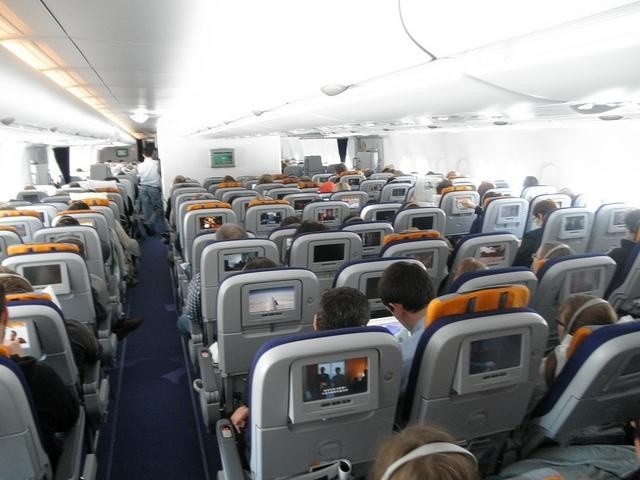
left=1, top=160, right=141, bottom=479
left=165, top=156, right=639, bottom=479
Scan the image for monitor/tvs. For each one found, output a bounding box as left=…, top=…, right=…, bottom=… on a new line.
left=374, top=208, right=398, bottom=225
left=358, top=271, right=407, bottom=308
left=229, top=183, right=293, bottom=220
left=424, top=178, right=441, bottom=191
left=496, top=202, right=521, bottom=224
left=607, top=209, right=634, bottom=233
left=239, top=278, right=304, bottom=328
left=455, top=185, right=477, bottom=192
left=558, top=266, right=606, bottom=300
left=308, top=239, right=350, bottom=272
left=408, top=213, right=438, bottom=230
left=115, top=148, right=128, bottom=157
left=451, top=195, right=474, bottom=214
left=317, top=170, right=412, bottom=194
left=605, top=348, right=640, bottom=388
left=292, top=197, right=316, bottom=214
left=0, top=191, right=115, bottom=259
left=195, top=212, right=227, bottom=236
left=218, top=246, right=265, bottom=283
left=256, top=209, right=287, bottom=233
left=314, top=206, right=343, bottom=228
left=0, top=317, right=44, bottom=363
left=340, top=194, right=362, bottom=213
left=390, top=187, right=408, bottom=202
left=356, top=229, right=385, bottom=255
left=210, top=149, right=235, bottom=167
left=553, top=200, right=562, bottom=210
left=558, top=214, right=588, bottom=239
left=16, top=261, right=71, bottom=300
left=400, top=247, right=439, bottom=278
left=474, top=242, right=511, bottom=266
left=288, top=348, right=380, bottom=424
left=452, top=327, right=532, bottom=395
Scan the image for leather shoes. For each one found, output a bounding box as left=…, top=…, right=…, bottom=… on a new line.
left=113, top=316, right=143, bottom=340
left=143, top=223, right=153, bottom=237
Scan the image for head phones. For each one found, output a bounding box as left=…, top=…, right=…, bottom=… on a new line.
left=564, top=298, right=608, bottom=335
left=534, top=244, right=569, bottom=266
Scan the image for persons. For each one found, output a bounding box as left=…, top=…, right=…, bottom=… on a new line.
left=136, top=146, right=161, bottom=236
